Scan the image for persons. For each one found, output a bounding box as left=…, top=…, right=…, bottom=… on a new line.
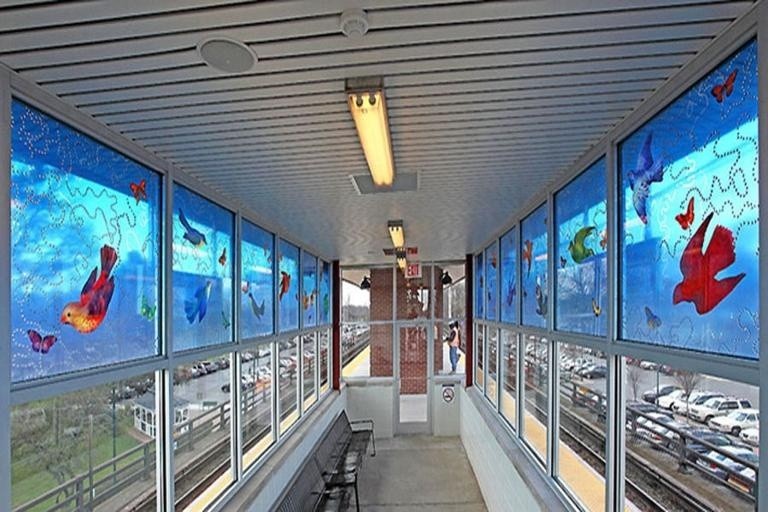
left=443, top=320, right=462, bottom=375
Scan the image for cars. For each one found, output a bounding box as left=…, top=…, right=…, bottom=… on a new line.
left=486, top=325, right=678, bottom=379
left=585, top=381, right=759, bottom=496
left=102, top=320, right=369, bottom=402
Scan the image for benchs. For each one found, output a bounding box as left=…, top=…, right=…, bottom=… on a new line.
left=267, top=407, right=376, bottom=512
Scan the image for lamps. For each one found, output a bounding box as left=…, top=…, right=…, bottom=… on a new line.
left=397, top=255, right=406, bottom=268
left=442, top=272, right=452, bottom=284
left=388, top=219, right=405, bottom=247
left=344, top=74, right=394, bottom=186
left=361, top=276, right=370, bottom=289
